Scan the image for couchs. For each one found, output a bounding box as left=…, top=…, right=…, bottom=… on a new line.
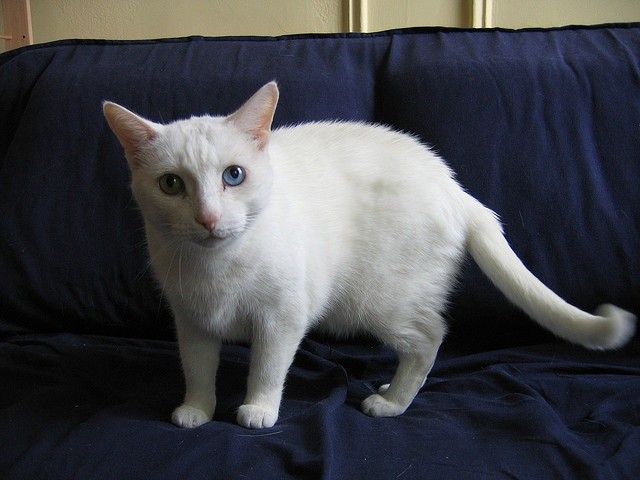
left=0, top=19, right=638, bottom=480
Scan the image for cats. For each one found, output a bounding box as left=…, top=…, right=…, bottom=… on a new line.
left=102, top=81, right=635, bottom=429
